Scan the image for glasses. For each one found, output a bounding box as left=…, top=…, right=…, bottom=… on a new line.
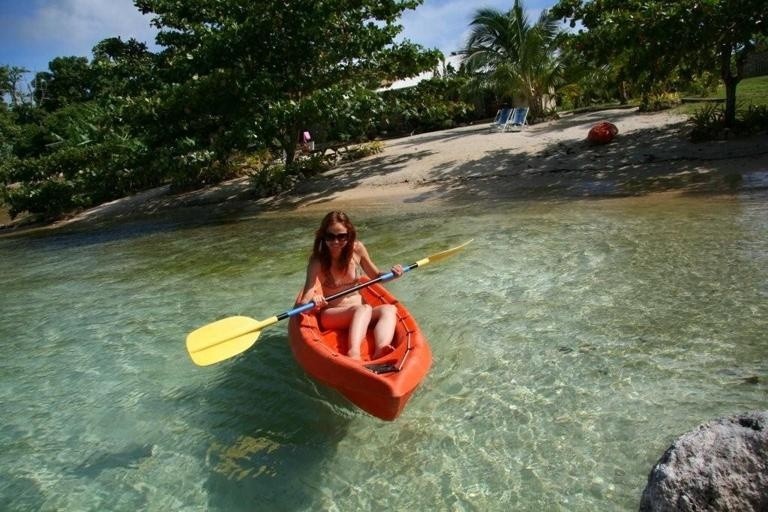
left=321, top=232, right=349, bottom=242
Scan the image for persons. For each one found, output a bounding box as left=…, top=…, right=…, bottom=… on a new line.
left=299, top=211, right=404, bottom=363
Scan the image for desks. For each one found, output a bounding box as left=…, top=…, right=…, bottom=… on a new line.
left=310, top=143, right=354, bottom=169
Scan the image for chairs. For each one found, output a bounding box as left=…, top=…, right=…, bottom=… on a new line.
left=490, top=108, right=514, bottom=133
left=506, top=106, right=531, bottom=133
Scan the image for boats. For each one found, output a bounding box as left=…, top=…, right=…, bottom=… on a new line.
left=288, top=275, right=432, bottom=422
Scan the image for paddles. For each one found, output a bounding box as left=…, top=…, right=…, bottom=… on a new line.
left=186, top=238, right=475, bottom=367
